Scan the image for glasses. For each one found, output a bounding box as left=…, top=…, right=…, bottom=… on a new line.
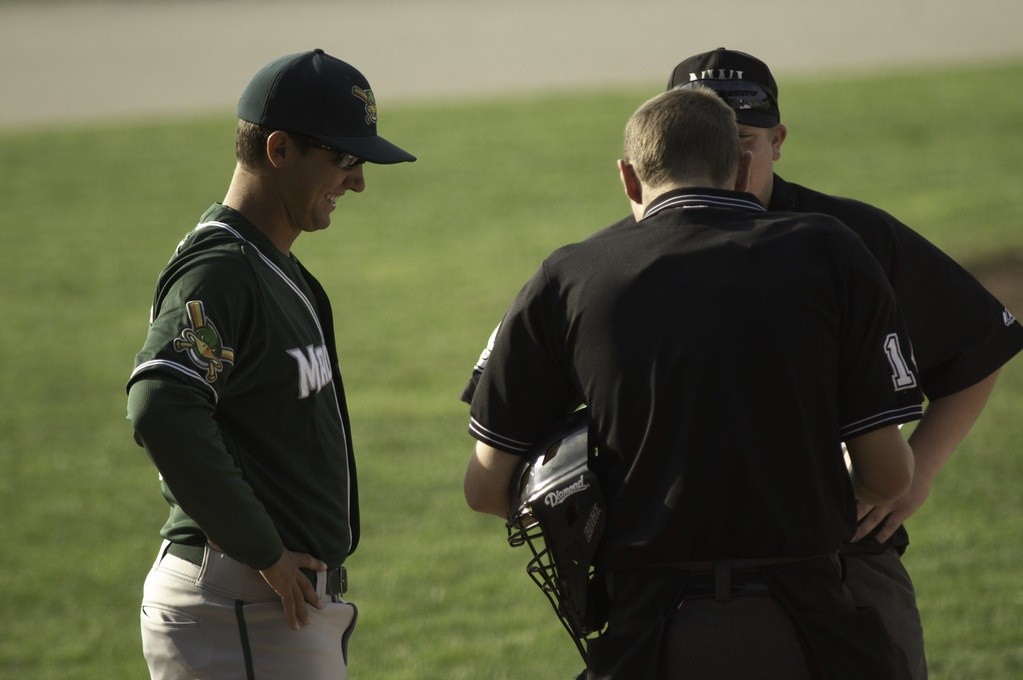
left=304, top=137, right=366, bottom=171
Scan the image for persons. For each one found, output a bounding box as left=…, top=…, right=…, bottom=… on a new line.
left=126, top=49, right=416, bottom=680
left=464, top=90, right=923, bottom=680
left=460, top=48, right=1023, bottom=680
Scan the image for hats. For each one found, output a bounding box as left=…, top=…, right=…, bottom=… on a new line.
left=236, top=48, right=417, bottom=165
left=666, top=46, right=780, bottom=128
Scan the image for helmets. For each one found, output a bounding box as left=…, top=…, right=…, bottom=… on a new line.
left=505, top=417, right=608, bottom=665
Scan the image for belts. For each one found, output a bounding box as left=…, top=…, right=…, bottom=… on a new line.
left=166, top=540, right=347, bottom=597
left=670, top=569, right=772, bottom=598
